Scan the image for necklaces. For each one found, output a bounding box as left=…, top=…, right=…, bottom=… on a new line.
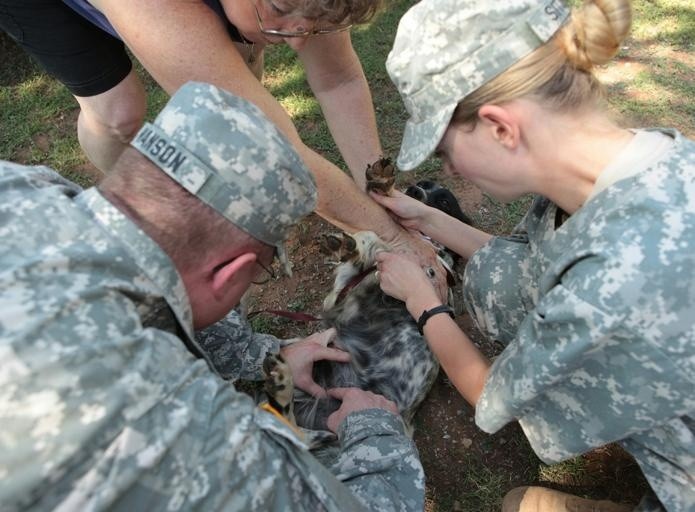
left=236, top=29, right=258, bottom=66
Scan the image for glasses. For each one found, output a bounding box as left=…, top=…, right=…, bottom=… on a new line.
left=250, top=3, right=355, bottom=40
left=211, top=252, right=277, bottom=285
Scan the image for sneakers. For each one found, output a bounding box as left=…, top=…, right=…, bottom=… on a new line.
left=500, top=487, right=628, bottom=512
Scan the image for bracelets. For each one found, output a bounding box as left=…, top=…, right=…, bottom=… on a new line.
left=417, top=304, right=457, bottom=336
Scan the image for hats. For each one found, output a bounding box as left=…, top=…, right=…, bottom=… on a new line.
left=129, top=80, right=319, bottom=278
left=384, top=0, right=572, bottom=174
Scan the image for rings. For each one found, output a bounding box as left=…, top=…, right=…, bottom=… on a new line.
left=426, top=267, right=436, bottom=279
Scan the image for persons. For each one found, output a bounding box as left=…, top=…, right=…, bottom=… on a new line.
left=0, top=78, right=429, bottom=512
left=367, top=0, right=695, bottom=512
left=0, top=1, right=448, bottom=308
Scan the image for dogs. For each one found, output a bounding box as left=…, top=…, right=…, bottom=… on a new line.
left=250, top=155, right=477, bottom=441
